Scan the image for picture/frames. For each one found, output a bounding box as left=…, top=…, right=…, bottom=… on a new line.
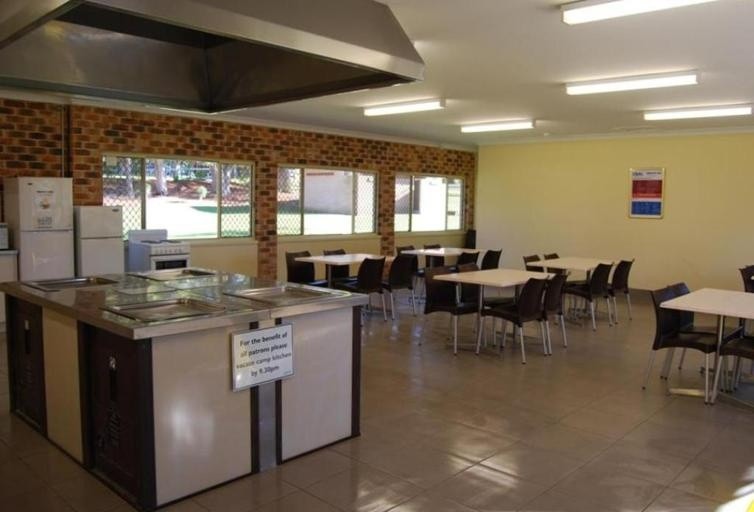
left=628, top=167, right=665, bottom=219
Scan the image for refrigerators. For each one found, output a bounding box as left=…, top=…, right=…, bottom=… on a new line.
left=73, top=206, right=124, bottom=277
left=3, top=177, right=76, bottom=281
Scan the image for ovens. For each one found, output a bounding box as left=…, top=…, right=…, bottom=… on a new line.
left=128, top=247, right=192, bottom=272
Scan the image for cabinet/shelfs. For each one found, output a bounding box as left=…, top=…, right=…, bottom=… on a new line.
left=0, top=252, right=17, bottom=332
left=0, top=285, right=253, bottom=510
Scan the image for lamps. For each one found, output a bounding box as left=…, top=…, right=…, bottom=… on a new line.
left=561, top=0, right=723, bottom=26
left=640, top=101, right=751, bottom=121
left=459, top=117, right=537, bottom=134
left=565, top=70, right=698, bottom=97
left=364, top=97, right=448, bottom=116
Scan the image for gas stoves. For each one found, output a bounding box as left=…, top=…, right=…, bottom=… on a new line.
left=127, top=229, right=188, bottom=247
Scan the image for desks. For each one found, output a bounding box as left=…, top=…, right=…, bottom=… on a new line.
left=660, top=288, right=754, bottom=388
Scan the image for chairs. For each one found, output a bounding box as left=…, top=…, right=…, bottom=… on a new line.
left=641, top=281, right=739, bottom=404
left=710, top=265, right=754, bottom=405
